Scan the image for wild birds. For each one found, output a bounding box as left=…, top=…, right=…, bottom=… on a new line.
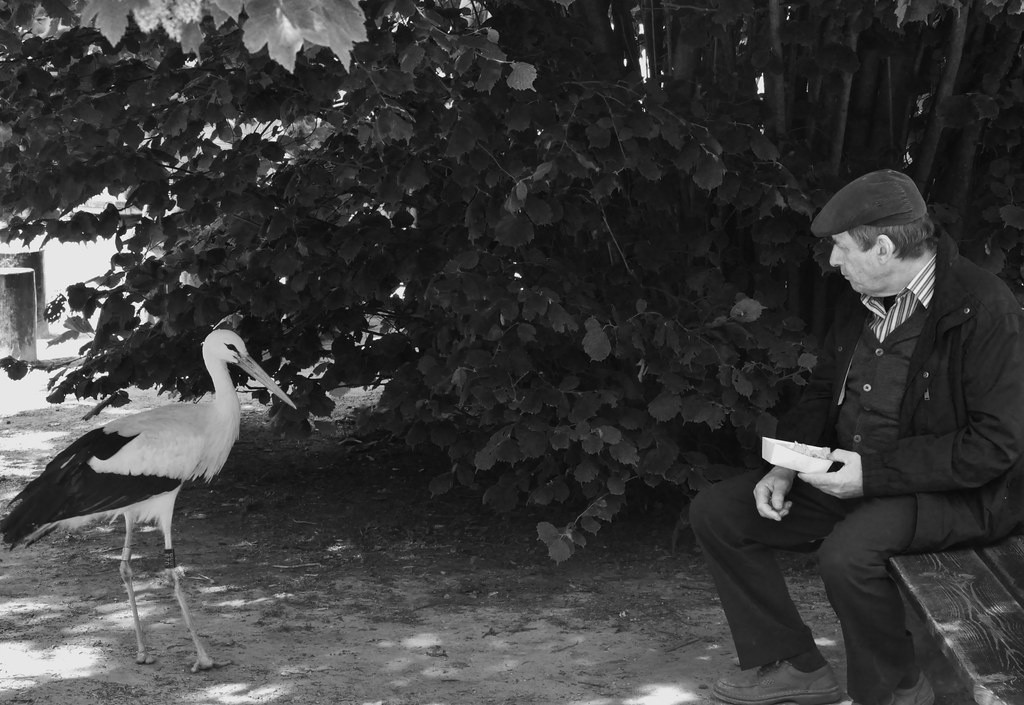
left=0, top=329, right=296, bottom=672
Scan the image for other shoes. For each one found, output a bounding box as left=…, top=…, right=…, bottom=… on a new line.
left=851, top=667, right=935, bottom=704
left=710, top=659, right=842, bottom=705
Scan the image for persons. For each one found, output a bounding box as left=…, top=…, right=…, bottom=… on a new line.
left=689, top=170, right=1024, bottom=705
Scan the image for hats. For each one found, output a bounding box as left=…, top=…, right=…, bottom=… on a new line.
left=812, top=169, right=926, bottom=238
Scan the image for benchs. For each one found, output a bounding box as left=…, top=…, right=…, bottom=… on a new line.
left=887, top=533, right=1024, bottom=705
